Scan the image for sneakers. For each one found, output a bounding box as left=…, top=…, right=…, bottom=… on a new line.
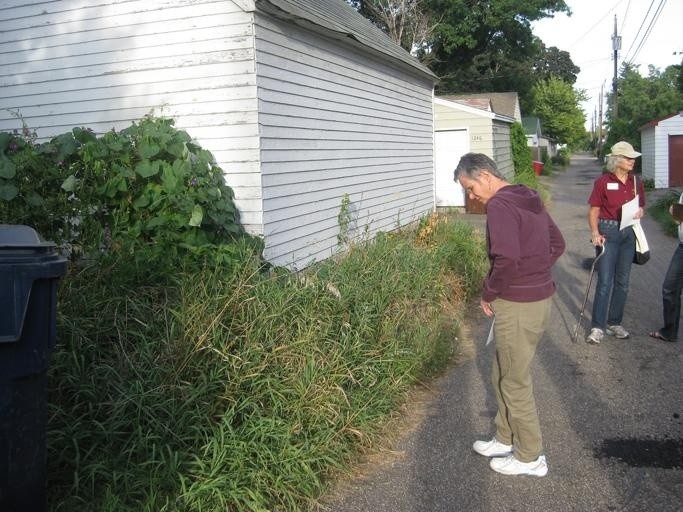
left=487, top=454, right=553, bottom=477
left=471, top=435, right=517, bottom=458
left=585, top=327, right=606, bottom=344
left=606, top=324, right=630, bottom=339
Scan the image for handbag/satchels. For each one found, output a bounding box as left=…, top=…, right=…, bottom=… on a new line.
left=630, top=222, right=651, bottom=265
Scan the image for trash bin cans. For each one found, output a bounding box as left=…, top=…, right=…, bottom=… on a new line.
left=533, top=161, right=545, bottom=176
left=0, top=224, right=70, bottom=512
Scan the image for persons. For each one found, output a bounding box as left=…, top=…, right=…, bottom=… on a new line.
left=586, top=140, right=646, bottom=344
left=451, top=150, right=567, bottom=477
left=648, top=192, right=683, bottom=342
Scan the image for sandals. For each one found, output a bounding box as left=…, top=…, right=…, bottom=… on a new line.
left=650, top=330, right=678, bottom=343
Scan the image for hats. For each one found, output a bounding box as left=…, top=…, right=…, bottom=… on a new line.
left=610, top=141, right=643, bottom=160
